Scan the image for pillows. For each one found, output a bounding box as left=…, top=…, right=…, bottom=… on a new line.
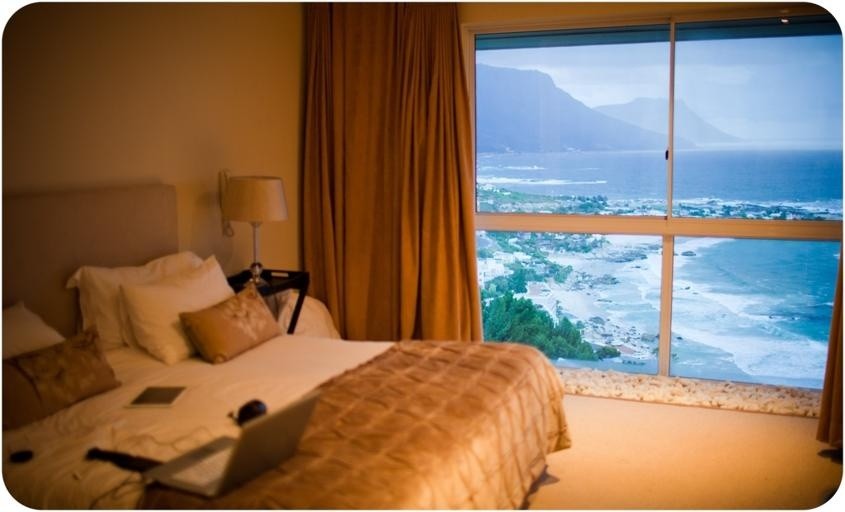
left=176, top=282, right=283, bottom=367
left=66, top=251, right=205, bottom=352
left=1, top=304, right=67, bottom=362
left=2, top=324, right=123, bottom=430
left=120, top=253, right=237, bottom=364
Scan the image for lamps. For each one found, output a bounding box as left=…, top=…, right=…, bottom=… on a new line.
left=221, top=175, right=289, bottom=289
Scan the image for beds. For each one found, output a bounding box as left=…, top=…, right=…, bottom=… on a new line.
left=3, top=182, right=573, bottom=510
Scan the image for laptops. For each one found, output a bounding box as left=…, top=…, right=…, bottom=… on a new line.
left=141, top=389, right=319, bottom=498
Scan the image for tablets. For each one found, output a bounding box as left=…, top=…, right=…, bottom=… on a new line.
left=129, top=385, right=188, bottom=407
